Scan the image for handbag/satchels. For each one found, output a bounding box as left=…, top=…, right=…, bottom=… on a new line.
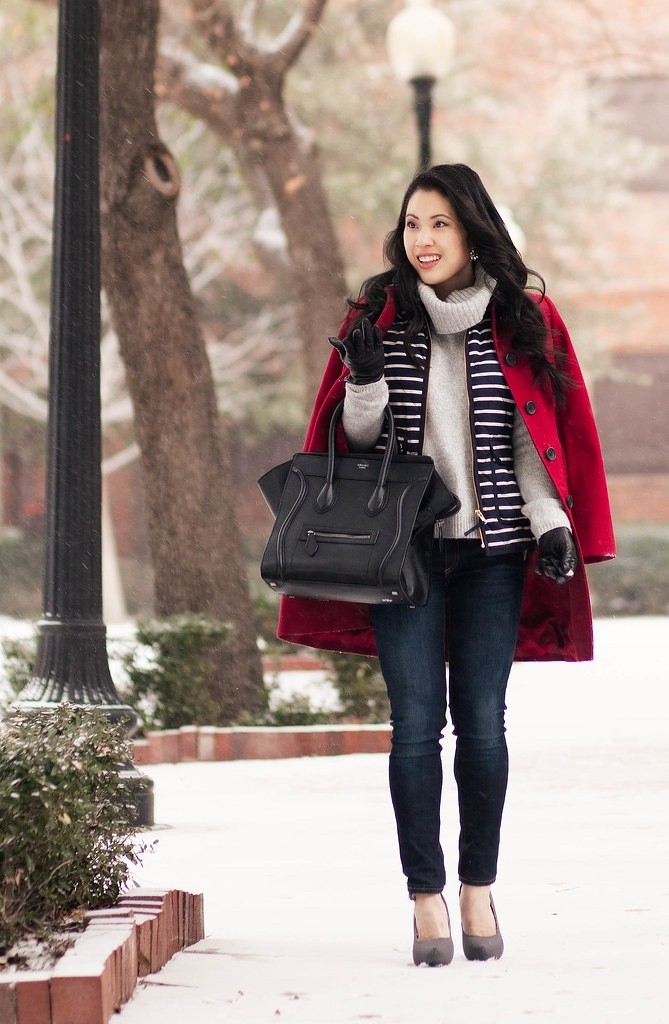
left=257, top=396, right=461, bottom=609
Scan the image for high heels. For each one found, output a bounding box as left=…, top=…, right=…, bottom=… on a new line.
left=459, top=882, right=504, bottom=961
left=413, top=892, right=454, bottom=967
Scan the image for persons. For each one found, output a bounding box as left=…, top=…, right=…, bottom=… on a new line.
left=277, top=163, right=616, bottom=966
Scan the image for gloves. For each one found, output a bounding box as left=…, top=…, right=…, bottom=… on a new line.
left=328, top=317, right=386, bottom=385
left=534, top=527, right=578, bottom=584
